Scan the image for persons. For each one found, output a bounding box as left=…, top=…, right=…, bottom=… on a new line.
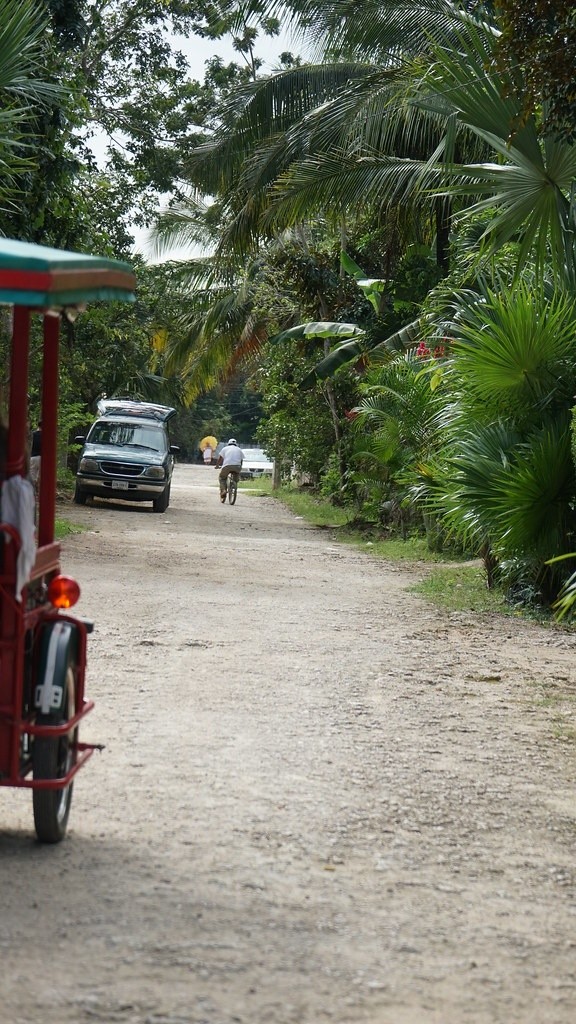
left=215, top=438, right=245, bottom=498
left=29, top=421, right=42, bottom=486
left=203, top=442, right=212, bottom=465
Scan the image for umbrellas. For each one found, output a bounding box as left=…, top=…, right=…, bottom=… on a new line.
left=200, top=436, right=218, bottom=451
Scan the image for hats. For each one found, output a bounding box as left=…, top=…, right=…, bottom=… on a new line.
left=228, top=439, right=237, bottom=445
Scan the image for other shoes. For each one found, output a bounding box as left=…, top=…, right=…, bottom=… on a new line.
left=231, top=482, right=235, bottom=488
left=220, top=491, right=226, bottom=498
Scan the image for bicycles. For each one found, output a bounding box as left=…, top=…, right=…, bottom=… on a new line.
left=215, top=466, right=237, bottom=505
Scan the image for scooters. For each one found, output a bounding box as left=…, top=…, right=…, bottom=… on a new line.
left=1, top=237, right=138, bottom=842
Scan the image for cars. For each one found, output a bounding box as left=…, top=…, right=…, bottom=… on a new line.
left=212, top=442, right=226, bottom=465
left=228, top=449, right=273, bottom=480
left=74, top=400, right=181, bottom=513
